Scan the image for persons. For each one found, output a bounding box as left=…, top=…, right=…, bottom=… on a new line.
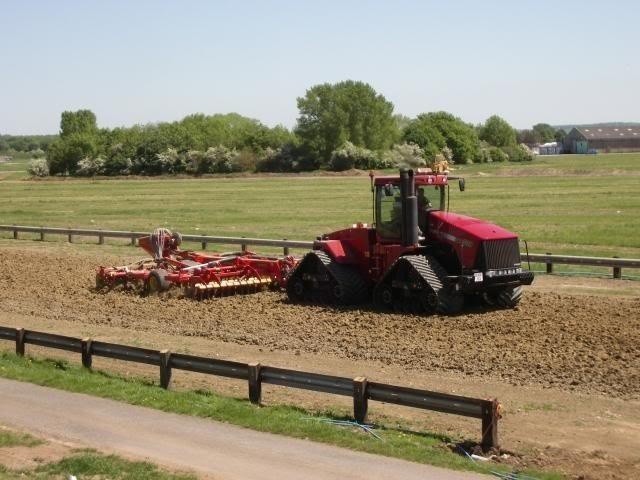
left=416, top=188, right=432, bottom=235
left=393, top=192, right=402, bottom=223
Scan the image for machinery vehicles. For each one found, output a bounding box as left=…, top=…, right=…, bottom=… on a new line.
left=416, top=157, right=450, bottom=175
left=280, top=165, right=534, bottom=311
